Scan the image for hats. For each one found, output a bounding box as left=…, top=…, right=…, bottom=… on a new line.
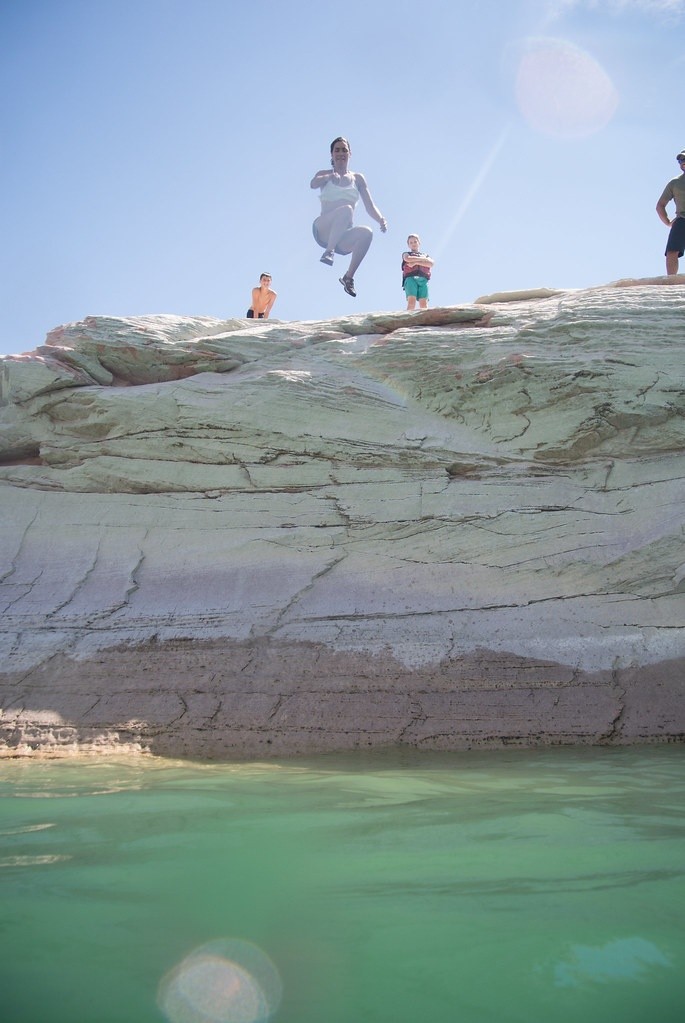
left=676, top=150, right=685, bottom=160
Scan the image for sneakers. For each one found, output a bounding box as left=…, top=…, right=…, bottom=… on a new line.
left=320, top=249, right=334, bottom=266
left=339, top=273, right=357, bottom=297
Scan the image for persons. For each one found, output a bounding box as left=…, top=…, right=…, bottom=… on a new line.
left=247, top=273, right=276, bottom=318
left=401, top=234, right=434, bottom=310
left=310, top=136, right=387, bottom=297
left=656, top=149, right=685, bottom=275
